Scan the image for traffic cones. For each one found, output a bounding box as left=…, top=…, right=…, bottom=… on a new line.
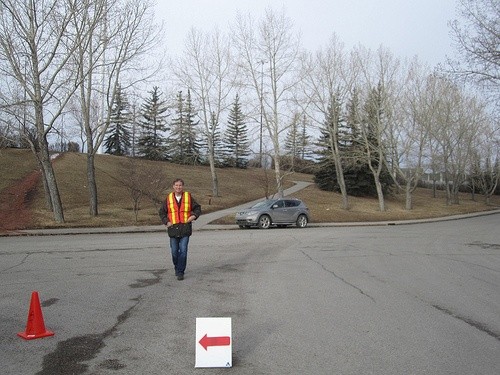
left=17, top=291, right=54, bottom=340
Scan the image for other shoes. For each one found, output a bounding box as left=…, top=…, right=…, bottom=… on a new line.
left=174, top=267, right=184, bottom=280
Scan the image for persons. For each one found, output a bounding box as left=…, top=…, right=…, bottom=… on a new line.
left=159, top=179, right=201, bottom=280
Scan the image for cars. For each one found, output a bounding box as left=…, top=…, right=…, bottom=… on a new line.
left=235, top=198, right=311, bottom=229
